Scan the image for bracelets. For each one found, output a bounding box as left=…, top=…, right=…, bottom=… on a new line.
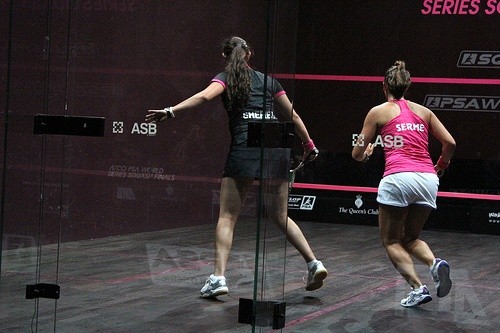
left=363, top=153, right=369, bottom=163
left=166, top=106, right=175, bottom=119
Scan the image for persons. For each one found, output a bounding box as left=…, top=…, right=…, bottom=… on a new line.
left=352, top=61, right=457, bottom=306
left=145, top=36, right=328, bottom=297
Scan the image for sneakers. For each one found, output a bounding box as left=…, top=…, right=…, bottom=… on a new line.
left=304, top=260, right=328, bottom=291
left=200, top=273, right=229, bottom=298
left=401, top=285, right=432, bottom=308
left=429, top=258, right=453, bottom=298
